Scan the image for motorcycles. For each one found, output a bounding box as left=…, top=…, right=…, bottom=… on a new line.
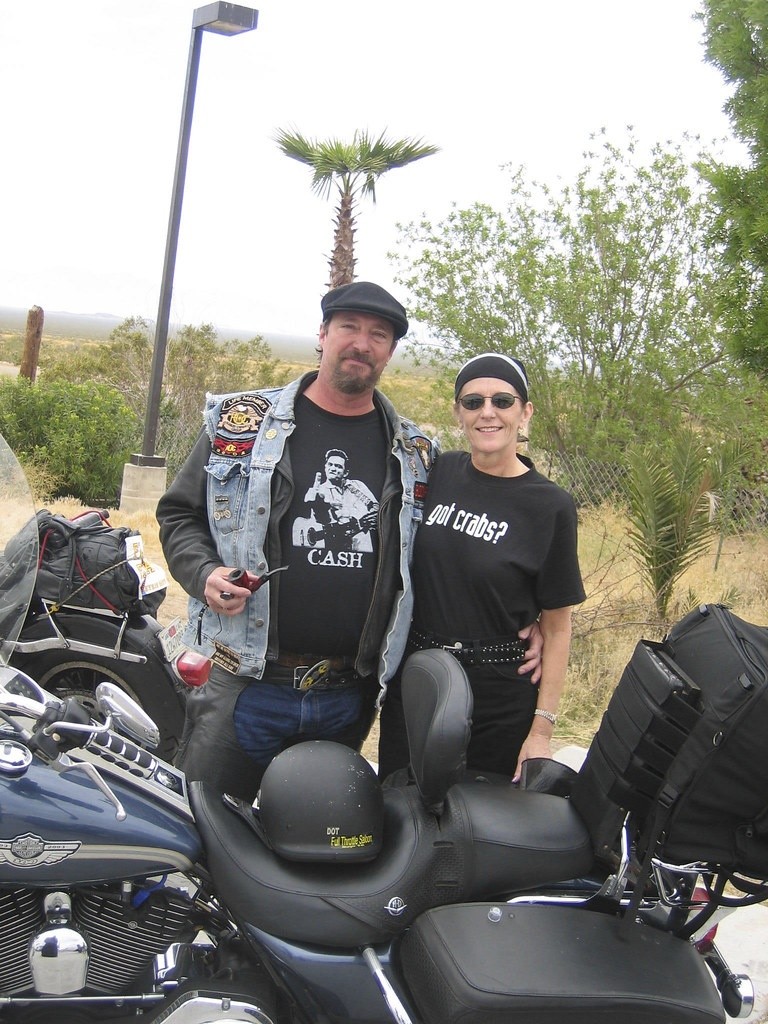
left=1, top=435, right=768, bottom=1024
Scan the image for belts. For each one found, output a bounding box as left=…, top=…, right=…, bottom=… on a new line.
left=410, top=625, right=528, bottom=666
left=272, top=649, right=357, bottom=692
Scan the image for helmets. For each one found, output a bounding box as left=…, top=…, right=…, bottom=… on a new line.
left=254, top=741, right=388, bottom=861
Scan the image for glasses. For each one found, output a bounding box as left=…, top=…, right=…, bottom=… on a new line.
left=457, top=391, right=525, bottom=410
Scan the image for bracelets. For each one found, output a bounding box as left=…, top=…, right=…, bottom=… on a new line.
left=536, top=613, right=541, bottom=623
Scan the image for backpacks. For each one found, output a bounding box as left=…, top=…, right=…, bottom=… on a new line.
left=568, top=601, right=768, bottom=882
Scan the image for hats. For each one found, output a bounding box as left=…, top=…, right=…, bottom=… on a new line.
left=455, top=353, right=528, bottom=402
left=321, top=282, right=408, bottom=338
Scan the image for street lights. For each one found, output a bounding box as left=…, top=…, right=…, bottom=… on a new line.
left=128, top=1, right=260, bottom=465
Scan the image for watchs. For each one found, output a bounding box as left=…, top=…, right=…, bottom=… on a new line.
left=534, top=709, right=557, bottom=724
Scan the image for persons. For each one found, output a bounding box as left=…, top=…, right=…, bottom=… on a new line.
left=378, top=353, right=572, bottom=783
left=156, top=282, right=544, bottom=806
